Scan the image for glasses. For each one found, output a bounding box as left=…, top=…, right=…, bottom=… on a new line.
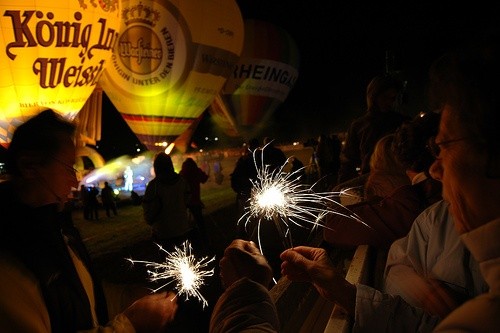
left=429, top=134, right=484, bottom=159
left=376, top=93, right=401, bottom=102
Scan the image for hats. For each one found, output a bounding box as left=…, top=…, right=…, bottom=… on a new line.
left=154, top=153, right=173, bottom=175
left=5, top=109, right=76, bottom=177
left=366, top=75, right=407, bottom=101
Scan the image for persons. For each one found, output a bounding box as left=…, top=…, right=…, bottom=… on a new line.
left=0, top=73, right=500, bottom=333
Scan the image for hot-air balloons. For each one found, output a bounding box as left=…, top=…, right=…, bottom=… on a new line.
left=0, top=0, right=123, bottom=151
left=98, top=0, right=245, bottom=161
left=206, top=20, right=300, bottom=150
